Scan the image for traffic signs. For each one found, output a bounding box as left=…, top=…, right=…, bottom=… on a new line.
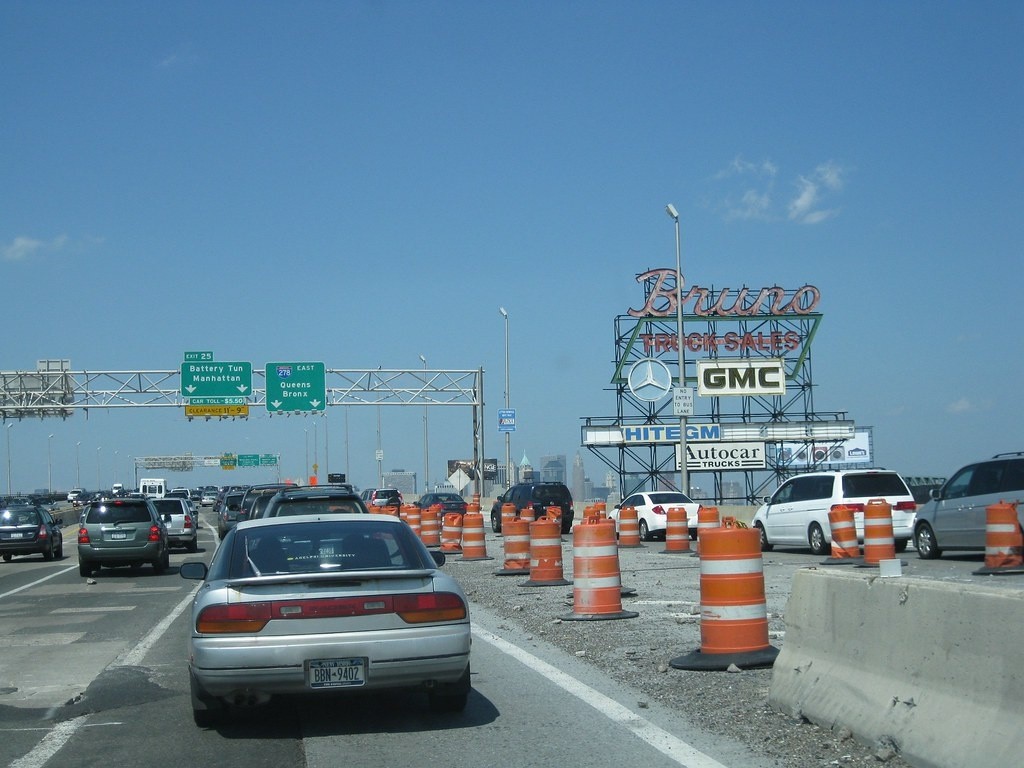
left=265, top=362, right=326, bottom=411
left=181, top=362, right=252, bottom=397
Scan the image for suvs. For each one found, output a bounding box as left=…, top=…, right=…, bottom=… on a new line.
left=491, top=482, right=574, bottom=535
left=751, top=466, right=917, bottom=553
left=912, top=451, right=1024, bottom=561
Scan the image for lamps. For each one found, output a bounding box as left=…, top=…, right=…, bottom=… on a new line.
left=188, top=413, right=249, bottom=422
left=277, top=409, right=316, bottom=417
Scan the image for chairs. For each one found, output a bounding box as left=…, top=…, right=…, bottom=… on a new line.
left=357, top=538, right=389, bottom=567
left=251, top=551, right=290, bottom=573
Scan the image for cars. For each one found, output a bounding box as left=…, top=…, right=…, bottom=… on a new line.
left=0, top=477, right=473, bottom=733
left=606, top=491, right=704, bottom=541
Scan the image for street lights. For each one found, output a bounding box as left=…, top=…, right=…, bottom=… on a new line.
left=342, top=400, right=349, bottom=485
left=499, top=306, right=511, bottom=490
left=76, top=441, right=81, bottom=488
left=47, top=433, right=55, bottom=494
left=97, top=447, right=131, bottom=490
left=376, top=365, right=383, bottom=490
left=312, top=421, right=317, bottom=477
left=417, top=353, right=428, bottom=495
left=304, top=429, right=308, bottom=483
left=663, top=202, right=691, bottom=500
left=323, top=414, right=329, bottom=474
left=6, top=423, right=13, bottom=496
